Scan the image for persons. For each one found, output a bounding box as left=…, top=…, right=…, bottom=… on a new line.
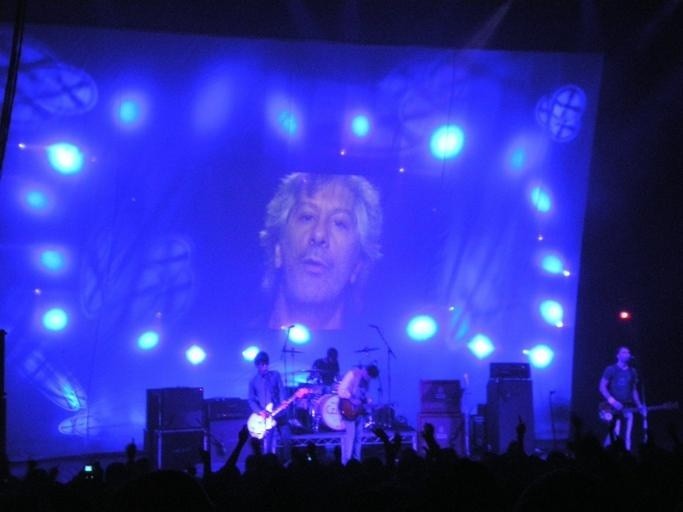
left=255, top=168, right=387, bottom=333
left=306, top=346, right=342, bottom=386
left=247, top=350, right=288, bottom=455
left=335, top=363, right=379, bottom=466
left=1, top=411, right=682, bottom=512
left=597, top=346, right=647, bottom=453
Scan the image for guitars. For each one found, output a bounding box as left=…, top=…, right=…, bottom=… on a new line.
left=246, top=388, right=313, bottom=440
left=338, top=393, right=378, bottom=420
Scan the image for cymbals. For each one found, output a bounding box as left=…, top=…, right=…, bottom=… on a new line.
left=354, top=347, right=379, bottom=353
left=283, top=349, right=300, bottom=353
left=302, top=369, right=324, bottom=373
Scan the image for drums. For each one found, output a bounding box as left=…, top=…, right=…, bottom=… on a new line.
left=314, top=393, right=346, bottom=432
left=296, top=382, right=315, bottom=399
left=330, top=382, right=340, bottom=393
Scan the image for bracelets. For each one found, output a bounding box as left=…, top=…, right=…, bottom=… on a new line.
left=607, top=396, right=616, bottom=406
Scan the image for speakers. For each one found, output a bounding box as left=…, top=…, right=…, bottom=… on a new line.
left=417, top=413, right=467, bottom=455
left=146, top=388, right=204, bottom=429
left=419, top=379, right=460, bottom=414
left=144, top=428, right=209, bottom=480
left=486, top=378, right=535, bottom=456
